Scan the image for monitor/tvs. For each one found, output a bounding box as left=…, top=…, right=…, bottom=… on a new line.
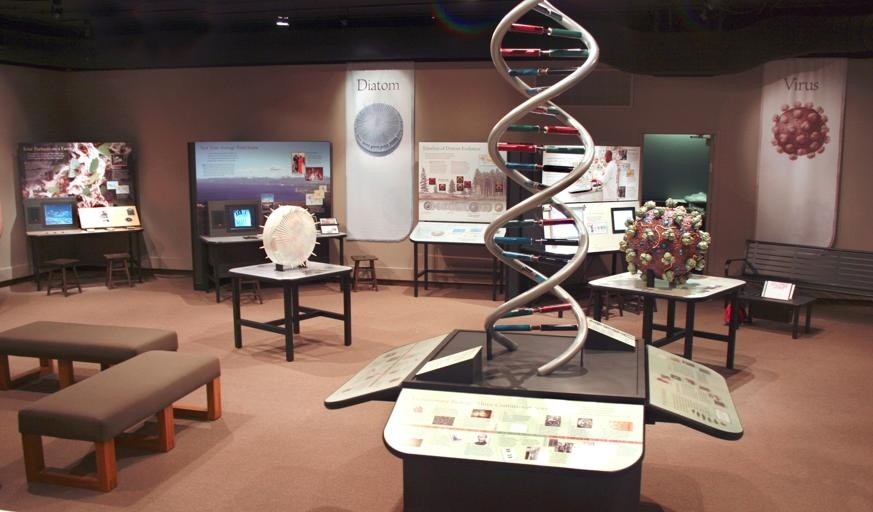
left=226, top=204, right=260, bottom=236
left=39, top=199, right=78, bottom=230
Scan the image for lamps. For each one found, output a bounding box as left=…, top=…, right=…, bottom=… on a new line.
left=276, top=15, right=290, bottom=26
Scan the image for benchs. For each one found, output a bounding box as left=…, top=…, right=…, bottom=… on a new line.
left=724, top=239, right=873, bottom=309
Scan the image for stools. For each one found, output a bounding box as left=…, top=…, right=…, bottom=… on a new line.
left=585, top=276, right=623, bottom=320
left=239, top=278, right=262, bottom=304
left=351, top=255, right=378, bottom=291
left=44, top=258, right=82, bottom=297
left=104, top=252, right=131, bottom=289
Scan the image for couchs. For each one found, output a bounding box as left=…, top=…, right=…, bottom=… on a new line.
left=17, top=349, right=222, bottom=492
left=0, top=320, right=178, bottom=393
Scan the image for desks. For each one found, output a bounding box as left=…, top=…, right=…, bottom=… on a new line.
left=324, top=328, right=742, bottom=511
left=199, top=228, right=347, bottom=304
left=229, top=261, right=353, bottom=362
left=25, top=226, right=144, bottom=291
left=588, top=268, right=747, bottom=370
